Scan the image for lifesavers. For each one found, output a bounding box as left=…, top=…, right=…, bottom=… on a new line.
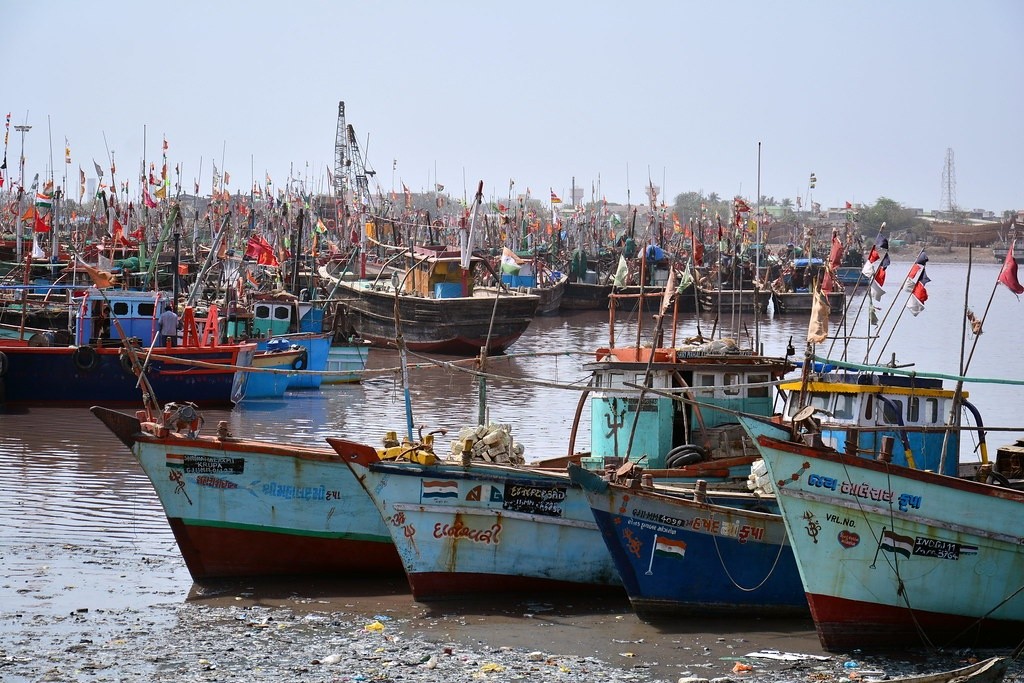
left=121, top=347, right=152, bottom=376
left=71, top=345, right=99, bottom=374
left=0, top=351, right=8, bottom=379
left=291, top=356, right=308, bottom=370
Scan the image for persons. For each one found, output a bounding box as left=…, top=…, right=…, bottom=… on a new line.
left=158, top=305, right=178, bottom=347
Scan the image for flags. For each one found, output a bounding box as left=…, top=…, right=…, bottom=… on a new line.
left=0, top=110, right=852, bottom=317
left=805, top=221, right=1024, bottom=344
left=500, top=246, right=526, bottom=276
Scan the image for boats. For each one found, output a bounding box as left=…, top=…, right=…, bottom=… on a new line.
left=992, top=235, right=1024, bottom=264
left=618, top=377, right=1024, bottom=656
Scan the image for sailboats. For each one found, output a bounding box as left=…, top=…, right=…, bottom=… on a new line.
left=0, top=97, right=968, bottom=621
